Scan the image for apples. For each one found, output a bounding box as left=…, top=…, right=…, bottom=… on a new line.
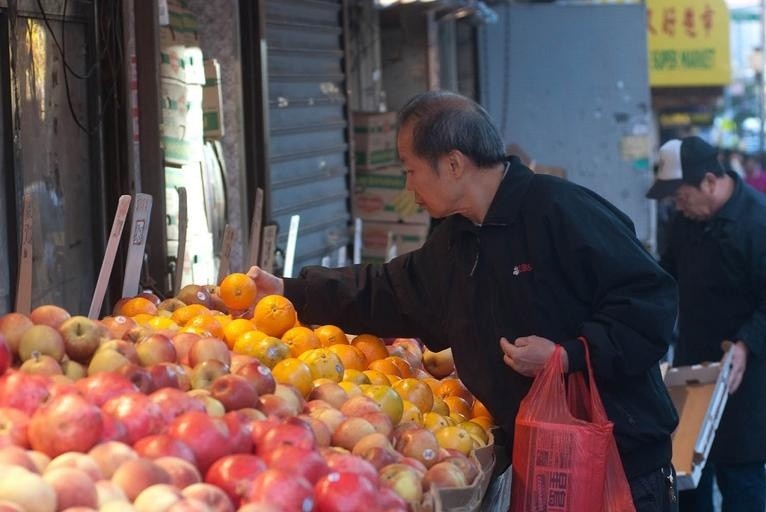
left=0, top=303, right=476, bottom=512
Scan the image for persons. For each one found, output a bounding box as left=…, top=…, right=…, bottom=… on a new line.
left=643, top=136, right=766, bottom=511
left=715, top=144, right=766, bottom=195
left=244, top=90, right=680, bottom=511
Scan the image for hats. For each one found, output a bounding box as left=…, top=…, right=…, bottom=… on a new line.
left=645, top=136, right=718, bottom=199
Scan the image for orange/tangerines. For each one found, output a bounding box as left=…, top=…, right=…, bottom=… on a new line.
left=220, top=272, right=257, bottom=310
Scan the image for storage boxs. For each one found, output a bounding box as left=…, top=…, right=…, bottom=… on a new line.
left=345, top=106, right=431, bottom=275
left=123, top=0, right=238, bottom=298
